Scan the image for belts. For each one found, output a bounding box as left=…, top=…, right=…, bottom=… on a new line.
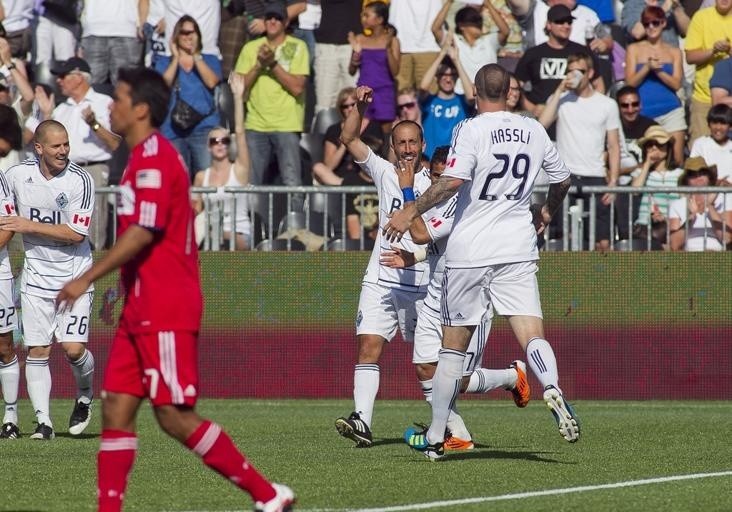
left=77, top=161, right=106, bottom=166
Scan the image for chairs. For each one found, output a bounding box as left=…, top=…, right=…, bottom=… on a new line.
left=616, top=238, right=663, bottom=249
left=169, top=82, right=376, bottom=253
left=545, top=238, right=588, bottom=250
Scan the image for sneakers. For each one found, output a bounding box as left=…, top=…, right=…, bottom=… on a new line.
left=255, top=484, right=296, bottom=512
left=508, top=360, right=530, bottom=407
left=443, top=435, right=475, bottom=451
left=404, top=426, right=446, bottom=462
left=69, top=396, right=92, bottom=435
left=541, top=384, right=582, bottom=443
left=30, top=420, right=57, bottom=440
left=334, top=413, right=372, bottom=447
left=0, top=422, right=20, bottom=440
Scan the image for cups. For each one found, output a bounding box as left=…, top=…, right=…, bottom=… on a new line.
left=566, top=69, right=584, bottom=90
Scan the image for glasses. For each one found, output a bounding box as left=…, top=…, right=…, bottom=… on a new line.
left=643, top=20, right=659, bottom=28
left=400, top=102, right=415, bottom=110
left=179, top=29, right=193, bottom=36
left=554, top=20, right=573, bottom=25
left=341, top=103, right=354, bottom=109
left=620, top=101, right=640, bottom=107
left=209, top=136, right=230, bottom=145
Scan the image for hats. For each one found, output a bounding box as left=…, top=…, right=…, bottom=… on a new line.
left=637, top=125, right=675, bottom=146
left=548, top=4, right=576, bottom=21
left=51, top=57, right=90, bottom=74
left=677, top=156, right=717, bottom=186
left=265, top=3, right=288, bottom=20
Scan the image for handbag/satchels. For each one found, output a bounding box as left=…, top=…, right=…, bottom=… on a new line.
left=633, top=222, right=667, bottom=242
left=171, top=97, right=203, bottom=130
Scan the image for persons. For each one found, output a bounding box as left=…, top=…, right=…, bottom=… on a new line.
left=391, top=141, right=533, bottom=452
left=0, top=167, right=23, bottom=440
left=0, top=118, right=96, bottom=441
left=0, top=0, right=732, bottom=251
left=381, top=62, right=583, bottom=464
left=50, top=65, right=298, bottom=512
left=331, top=84, right=431, bottom=448
